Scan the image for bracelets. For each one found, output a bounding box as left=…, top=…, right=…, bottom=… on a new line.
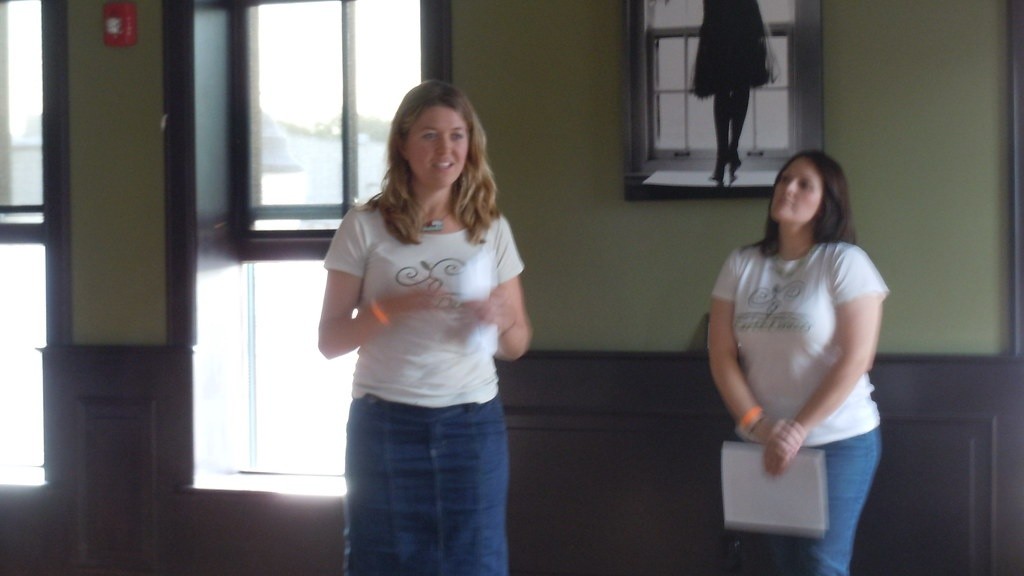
left=740, top=405, right=762, bottom=428
left=371, top=301, right=390, bottom=326
left=499, top=322, right=515, bottom=338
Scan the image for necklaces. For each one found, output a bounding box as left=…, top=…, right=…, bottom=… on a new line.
left=772, top=245, right=817, bottom=276
left=422, top=213, right=450, bottom=231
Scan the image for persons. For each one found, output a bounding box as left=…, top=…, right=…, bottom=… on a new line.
left=708, top=150, right=890, bottom=576
left=318, top=80, right=529, bottom=576
left=689, top=0, right=780, bottom=185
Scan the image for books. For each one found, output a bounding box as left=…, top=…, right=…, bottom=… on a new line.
left=721, top=440, right=827, bottom=537
